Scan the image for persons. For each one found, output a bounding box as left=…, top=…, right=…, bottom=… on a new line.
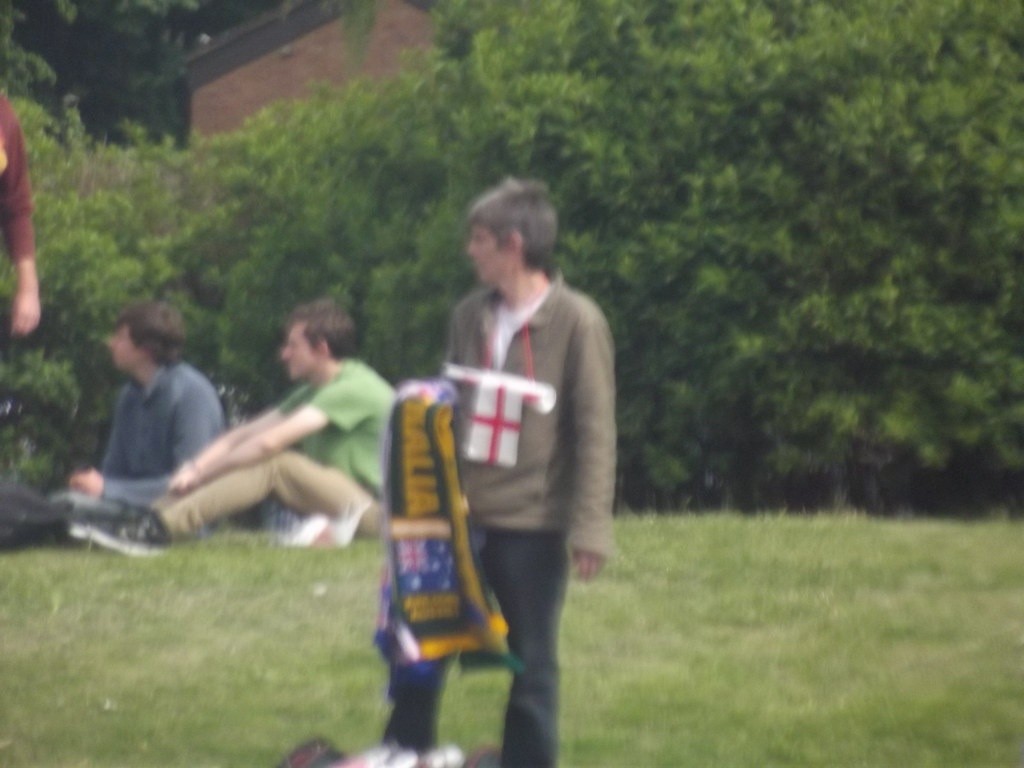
left=70, top=295, right=399, bottom=557
left=341, top=177, right=615, bottom=768
left=0, top=304, right=227, bottom=550
left=0, top=93, right=40, bottom=335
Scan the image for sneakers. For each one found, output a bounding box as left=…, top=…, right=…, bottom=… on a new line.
left=69, top=514, right=170, bottom=556
